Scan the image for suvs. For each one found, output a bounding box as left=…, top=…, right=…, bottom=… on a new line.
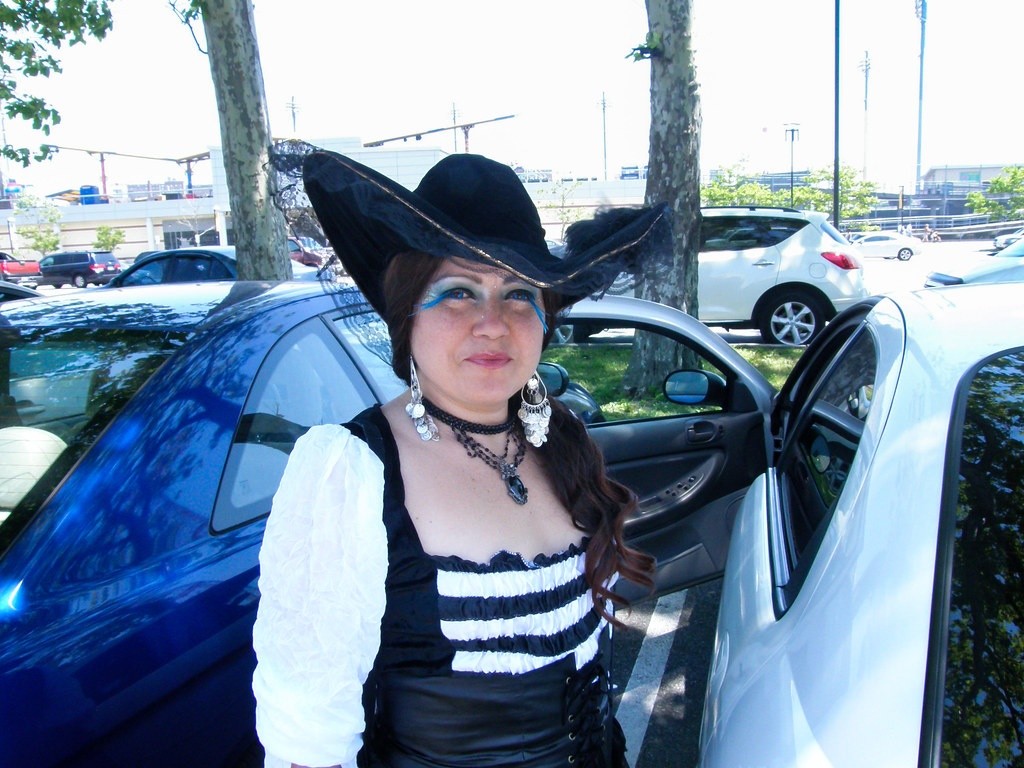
left=28, top=250, right=124, bottom=288
left=559, top=200, right=870, bottom=349
left=0, top=278, right=410, bottom=768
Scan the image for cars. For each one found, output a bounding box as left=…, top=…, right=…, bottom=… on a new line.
left=850, top=229, right=926, bottom=262
left=287, top=236, right=324, bottom=267
left=921, top=226, right=1024, bottom=289
left=533, top=282, right=1024, bottom=768
left=97, top=245, right=338, bottom=289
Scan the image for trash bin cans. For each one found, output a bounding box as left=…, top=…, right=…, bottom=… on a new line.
left=80, top=185, right=100, bottom=204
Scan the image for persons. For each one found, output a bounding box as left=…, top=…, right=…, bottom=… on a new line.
left=252, top=154, right=671, bottom=767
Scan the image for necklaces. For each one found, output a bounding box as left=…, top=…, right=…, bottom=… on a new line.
left=407, top=374, right=531, bottom=505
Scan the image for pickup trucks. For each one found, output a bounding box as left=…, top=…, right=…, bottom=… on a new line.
left=0, top=251, right=44, bottom=290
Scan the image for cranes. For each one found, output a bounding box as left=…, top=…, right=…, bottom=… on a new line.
left=362, top=114, right=515, bottom=153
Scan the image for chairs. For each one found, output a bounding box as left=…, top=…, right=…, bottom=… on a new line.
left=0, top=427, right=67, bottom=525
left=68, top=354, right=166, bottom=454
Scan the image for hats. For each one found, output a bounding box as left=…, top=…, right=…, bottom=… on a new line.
left=304, top=151, right=669, bottom=322
